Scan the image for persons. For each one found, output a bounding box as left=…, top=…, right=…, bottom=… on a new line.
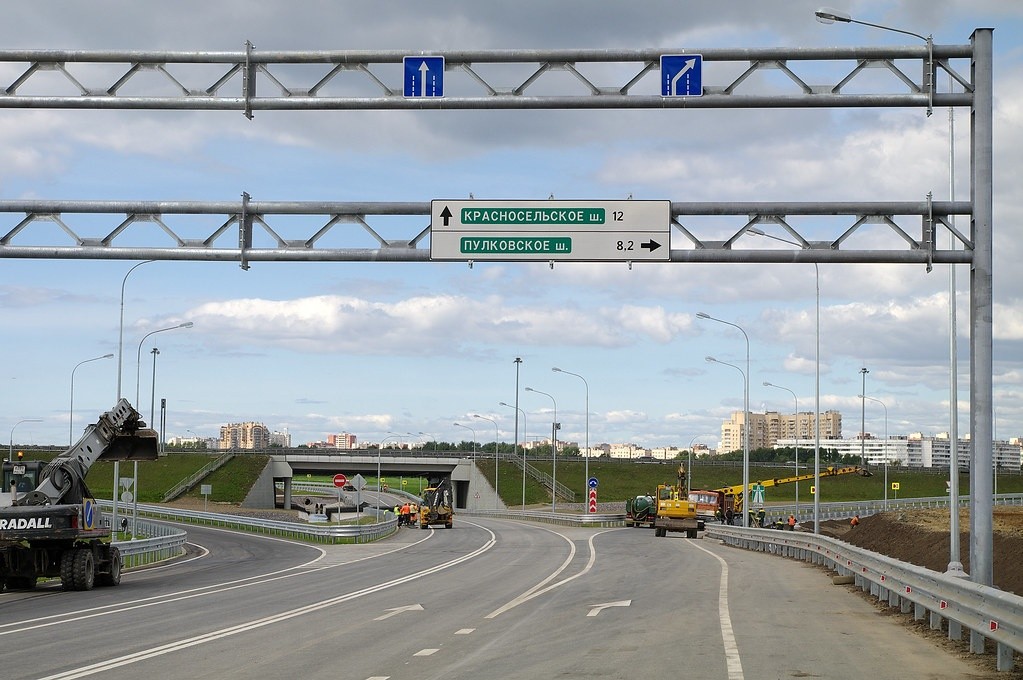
left=381, top=485, right=388, bottom=493
left=316, top=503, right=318, bottom=514
left=850, top=515, right=860, bottom=529
left=320, top=504, right=323, bottom=514
left=748, top=507, right=755, bottom=527
left=758, top=507, right=765, bottom=528
left=770, top=518, right=785, bottom=530
left=715, top=506, right=734, bottom=525
left=787, top=515, right=797, bottom=531
left=394, top=502, right=418, bottom=529
left=661, top=492, right=670, bottom=500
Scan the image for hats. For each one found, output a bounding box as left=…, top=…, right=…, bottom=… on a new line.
left=411, top=502, right=415, bottom=504
left=395, top=504, right=398, bottom=507
left=404, top=502, right=407, bottom=504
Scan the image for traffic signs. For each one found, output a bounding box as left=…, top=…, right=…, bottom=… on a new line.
left=430, top=198, right=671, bottom=265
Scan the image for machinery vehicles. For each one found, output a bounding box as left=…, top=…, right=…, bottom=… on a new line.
left=688, top=464, right=876, bottom=538
left=624, top=492, right=656, bottom=528
left=416, top=475, right=455, bottom=529
left=0, top=398, right=162, bottom=593
left=653, top=460, right=701, bottom=538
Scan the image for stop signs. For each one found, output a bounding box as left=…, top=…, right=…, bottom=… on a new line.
left=333, top=473, right=346, bottom=488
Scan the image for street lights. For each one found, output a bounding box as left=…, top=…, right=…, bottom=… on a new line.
left=687, top=433, right=714, bottom=498
left=10, top=419, right=49, bottom=465
left=454, top=423, right=476, bottom=460
left=408, top=432, right=423, bottom=452
left=419, top=431, right=436, bottom=450
left=552, top=367, right=591, bottom=515
left=376, top=436, right=409, bottom=523
left=136, top=321, right=194, bottom=412
left=513, top=357, right=518, bottom=454
left=69, top=352, right=114, bottom=450
left=474, top=415, right=499, bottom=510
left=746, top=226, right=820, bottom=536
left=150, top=347, right=158, bottom=430
left=500, top=402, right=527, bottom=516
left=762, top=381, right=800, bottom=517
left=524, top=387, right=557, bottom=514
left=696, top=311, right=751, bottom=529
left=813, top=7, right=968, bottom=577
left=858, top=393, right=886, bottom=505
left=860, top=366, right=873, bottom=467
